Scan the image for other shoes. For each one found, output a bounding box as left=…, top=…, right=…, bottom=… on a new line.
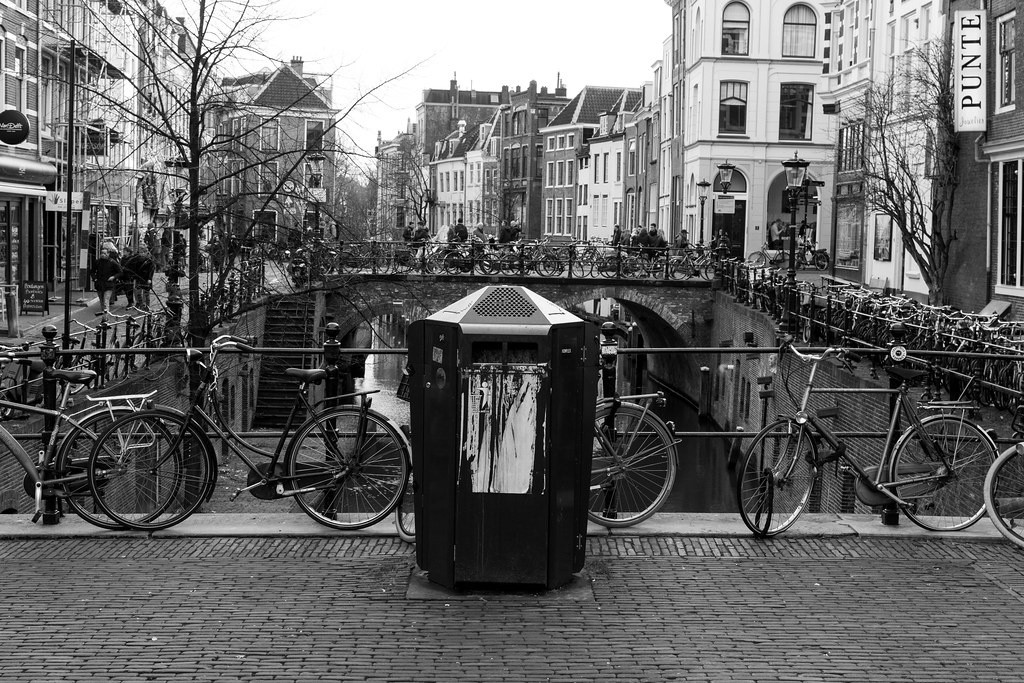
left=126, top=304, right=133, bottom=310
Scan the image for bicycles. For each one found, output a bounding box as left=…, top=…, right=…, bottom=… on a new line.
left=397, top=472, right=418, bottom=544
left=751, top=268, right=1024, bottom=415
left=739, top=334, right=997, bottom=539
left=88, top=333, right=412, bottom=530
left=1, top=350, right=183, bottom=530
left=748, top=242, right=794, bottom=269
left=793, top=236, right=830, bottom=270
left=983, top=404, right=1024, bottom=550
left=0, top=317, right=90, bottom=423
left=290, top=229, right=729, bottom=291
left=588, top=390, right=682, bottom=527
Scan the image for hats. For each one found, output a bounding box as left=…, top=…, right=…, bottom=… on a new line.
left=681, top=230, right=689, bottom=234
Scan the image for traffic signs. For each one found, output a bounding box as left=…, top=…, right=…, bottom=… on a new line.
left=794, top=180, right=825, bottom=206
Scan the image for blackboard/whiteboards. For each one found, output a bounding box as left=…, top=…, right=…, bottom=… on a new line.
left=20, top=280, right=48, bottom=312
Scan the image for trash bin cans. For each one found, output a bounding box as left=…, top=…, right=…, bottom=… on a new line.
left=405, top=284, right=594, bottom=597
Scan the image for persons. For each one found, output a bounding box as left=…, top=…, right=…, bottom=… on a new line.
left=798, top=220, right=816, bottom=244
left=611, top=223, right=732, bottom=278
left=768, top=218, right=790, bottom=250
left=90, top=223, right=187, bottom=317
left=447, top=217, right=522, bottom=274
left=403, top=222, right=432, bottom=249
left=324, top=215, right=337, bottom=242
left=198, top=230, right=240, bottom=272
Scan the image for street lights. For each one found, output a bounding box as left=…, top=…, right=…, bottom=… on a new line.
left=64, top=39, right=87, bottom=368
left=697, top=178, right=711, bottom=261
left=779, top=149, right=811, bottom=332
